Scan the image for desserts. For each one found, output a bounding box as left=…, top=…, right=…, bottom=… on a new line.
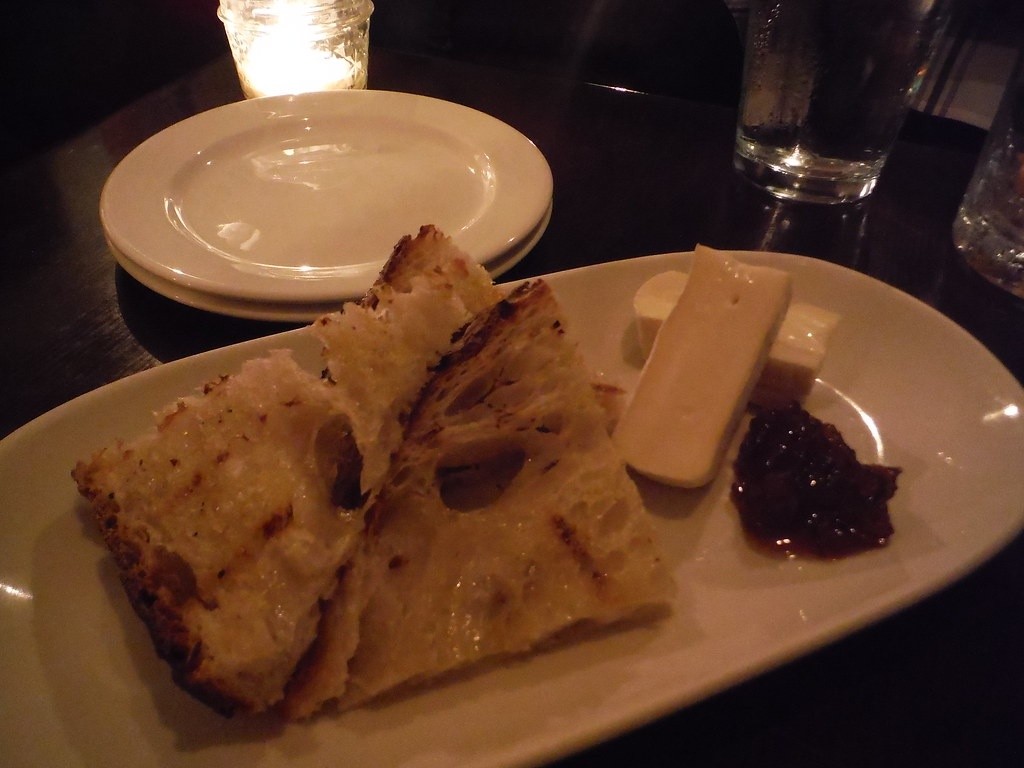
left=610, top=239, right=793, bottom=494
left=632, top=271, right=838, bottom=408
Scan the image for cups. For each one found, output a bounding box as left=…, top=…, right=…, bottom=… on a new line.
left=952, top=54, right=1024, bottom=302
left=217, top=0, right=376, bottom=105
left=728, top=1, right=958, bottom=205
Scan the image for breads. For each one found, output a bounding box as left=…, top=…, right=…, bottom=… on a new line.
left=68, top=224, right=680, bottom=720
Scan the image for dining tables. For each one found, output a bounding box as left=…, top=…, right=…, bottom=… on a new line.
left=2, top=42, right=1024, bottom=768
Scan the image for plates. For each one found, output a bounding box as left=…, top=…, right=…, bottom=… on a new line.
left=102, top=198, right=562, bottom=328
left=0, top=249, right=1024, bottom=768
left=98, top=90, right=555, bottom=305
